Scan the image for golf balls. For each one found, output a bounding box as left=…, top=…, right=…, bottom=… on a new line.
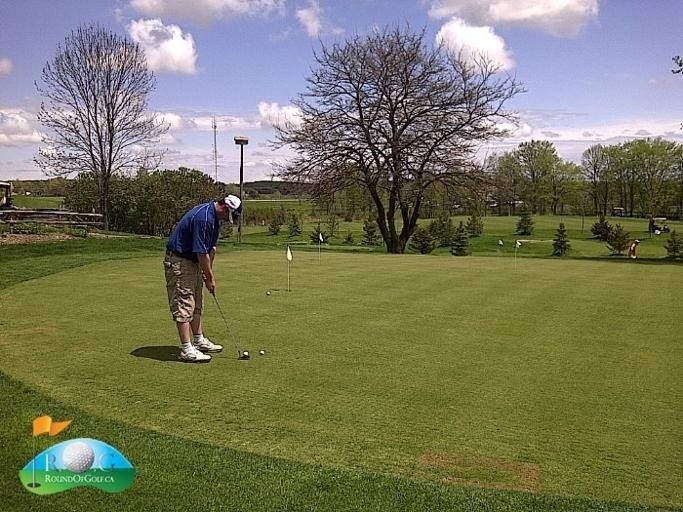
left=260, top=351, right=264, bottom=355
left=64, top=442, right=95, bottom=474
left=243, top=351, right=249, bottom=356
left=267, top=292, right=270, bottom=295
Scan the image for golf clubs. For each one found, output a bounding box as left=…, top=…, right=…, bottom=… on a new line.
left=203, top=276, right=250, bottom=360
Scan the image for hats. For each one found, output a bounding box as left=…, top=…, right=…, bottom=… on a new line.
left=223, top=194, right=242, bottom=226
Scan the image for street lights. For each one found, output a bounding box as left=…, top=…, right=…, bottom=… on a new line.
left=233, top=134, right=248, bottom=243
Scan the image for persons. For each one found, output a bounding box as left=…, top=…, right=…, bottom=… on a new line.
left=163, top=192, right=243, bottom=363
left=628, top=240, right=640, bottom=264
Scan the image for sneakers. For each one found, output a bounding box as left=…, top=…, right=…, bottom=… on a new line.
left=178, top=345, right=212, bottom=363
left=193, top=337, right=224, bottom=353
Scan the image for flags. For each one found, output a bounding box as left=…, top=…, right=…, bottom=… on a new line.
left=515, top=241, right=521, bottom=249
left=318, top=232, right=323, bottom=242
left=498, top=240, right=503, bottom=246
left=286, top=244, right=293, bottom=261
